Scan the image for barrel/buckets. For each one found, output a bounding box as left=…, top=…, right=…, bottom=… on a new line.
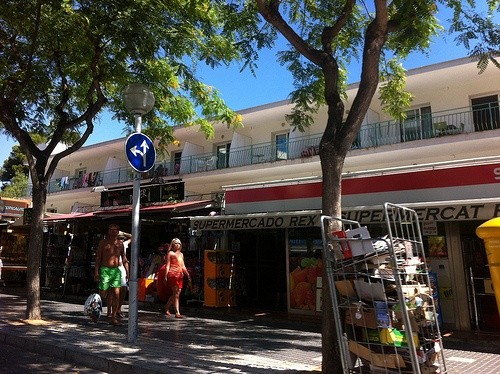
left=138, top=279, right=155, bottom=301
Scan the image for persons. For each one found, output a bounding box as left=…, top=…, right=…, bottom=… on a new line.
left=160, top=239, right=190, bottom=319
left=94, top=223, right=129, bottom=325
left=68, top=241, right=86, bottom=295
left=154, top=164, right=164, bottom=178
left=107, top=230, right=132, bottom=318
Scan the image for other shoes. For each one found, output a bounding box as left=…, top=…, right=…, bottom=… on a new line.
left=165, top=306, right=171, bottom=315
left=175, top=314, right=183, bottom=318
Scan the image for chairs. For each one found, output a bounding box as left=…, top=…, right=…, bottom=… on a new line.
left=433, top=122, right=464, bottom=138
left=195, top=156, right=218, bottom=172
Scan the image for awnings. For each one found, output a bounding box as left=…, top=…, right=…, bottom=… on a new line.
left=43, top=198, right=212, bottom=221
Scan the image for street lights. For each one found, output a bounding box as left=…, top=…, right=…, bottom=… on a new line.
left=122, top=82, right=156, bottom=344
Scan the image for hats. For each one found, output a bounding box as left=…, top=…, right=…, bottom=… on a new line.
left=117, top=233, right=126, bottom=239
